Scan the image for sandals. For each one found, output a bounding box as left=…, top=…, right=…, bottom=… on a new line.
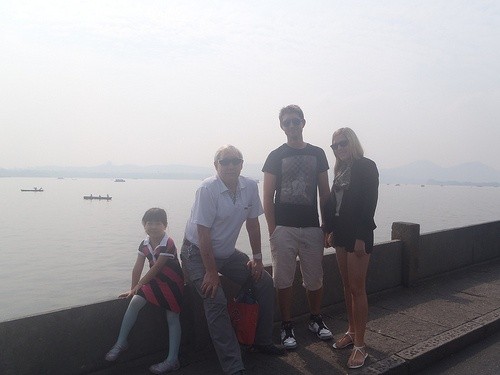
left=332, top=331, right=355, bottom=349
left=346, top=344, right=368, bottom=368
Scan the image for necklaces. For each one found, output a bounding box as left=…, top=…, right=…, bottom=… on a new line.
left=227, top=184, right=235, bottom=199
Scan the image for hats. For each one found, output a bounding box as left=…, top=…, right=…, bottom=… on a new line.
left=229, top=269, right=269, bottom=303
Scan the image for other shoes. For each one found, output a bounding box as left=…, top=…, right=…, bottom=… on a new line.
left=150, top=360, right=181, bottom=373
left=105, top=344, right=128, bottom=362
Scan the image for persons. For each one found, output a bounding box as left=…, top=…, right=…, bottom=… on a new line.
left=324, top=126, right=379, bottom=369
left=180, top=143, right=288, bottom=375
left=262, top=104, right=332, bottom=349
left=105, top=208, right=185, bottom=375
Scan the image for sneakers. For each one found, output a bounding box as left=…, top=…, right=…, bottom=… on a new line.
left=308, top=318, right=332, bottom=340
left=280, top=327, right=297, bottom=350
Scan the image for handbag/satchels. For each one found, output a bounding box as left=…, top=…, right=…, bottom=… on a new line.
left=230, top=304, right=268, bottom=344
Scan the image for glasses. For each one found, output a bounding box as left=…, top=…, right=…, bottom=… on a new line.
left=219, top=159, right=244, bottom=166
left=283, top=118, right=303, bottom=127
left=330, top=140, right=350, bottom=151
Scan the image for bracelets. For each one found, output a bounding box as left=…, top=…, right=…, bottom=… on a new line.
left=138, top=280, right=145, bottom=288
left=253, top=253, right=262, bottom=260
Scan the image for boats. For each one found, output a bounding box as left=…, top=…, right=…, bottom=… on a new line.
left=113, top=178, right=125, bottom=182
